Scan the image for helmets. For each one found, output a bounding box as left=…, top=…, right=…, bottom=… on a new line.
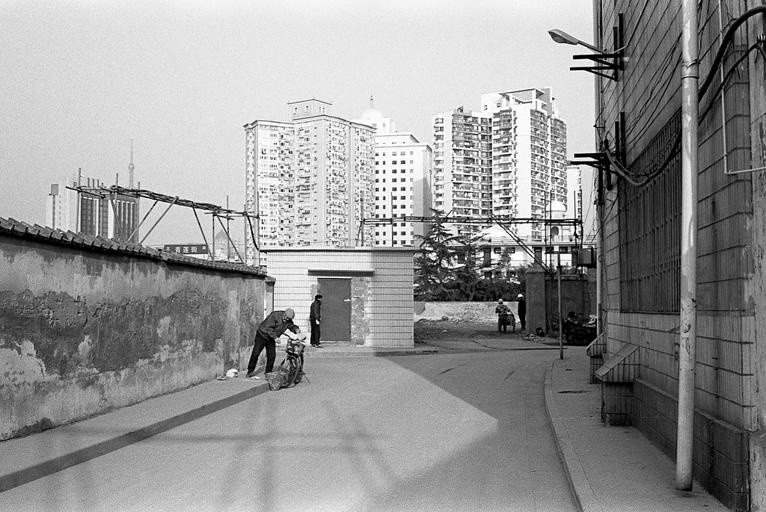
left=518, top=294, right=523, bottom=298
left=499, top=299, right=503, bottom=304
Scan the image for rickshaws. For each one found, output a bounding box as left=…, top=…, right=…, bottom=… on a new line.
left=507, top=310, right=516, bottom=333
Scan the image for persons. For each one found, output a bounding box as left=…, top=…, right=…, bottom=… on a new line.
left=518, top=294, right=526, bottom=331
left=495, top=298, right=510, bottom=333
left=246, top=308, right=301, bottom=377
left=310, top=295, right=322, bottom=348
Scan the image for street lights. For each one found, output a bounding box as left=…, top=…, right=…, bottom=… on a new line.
left=544, top=246, right=580, bottom=359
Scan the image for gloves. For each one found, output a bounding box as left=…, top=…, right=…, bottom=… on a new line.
left=316, top=320, right=320, bottom=324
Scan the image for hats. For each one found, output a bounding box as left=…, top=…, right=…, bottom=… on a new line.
left=285, top=308, right=295, bottom=319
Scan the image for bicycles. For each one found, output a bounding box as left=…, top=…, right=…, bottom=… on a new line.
left=278, top=330, right=311, bottom=388
